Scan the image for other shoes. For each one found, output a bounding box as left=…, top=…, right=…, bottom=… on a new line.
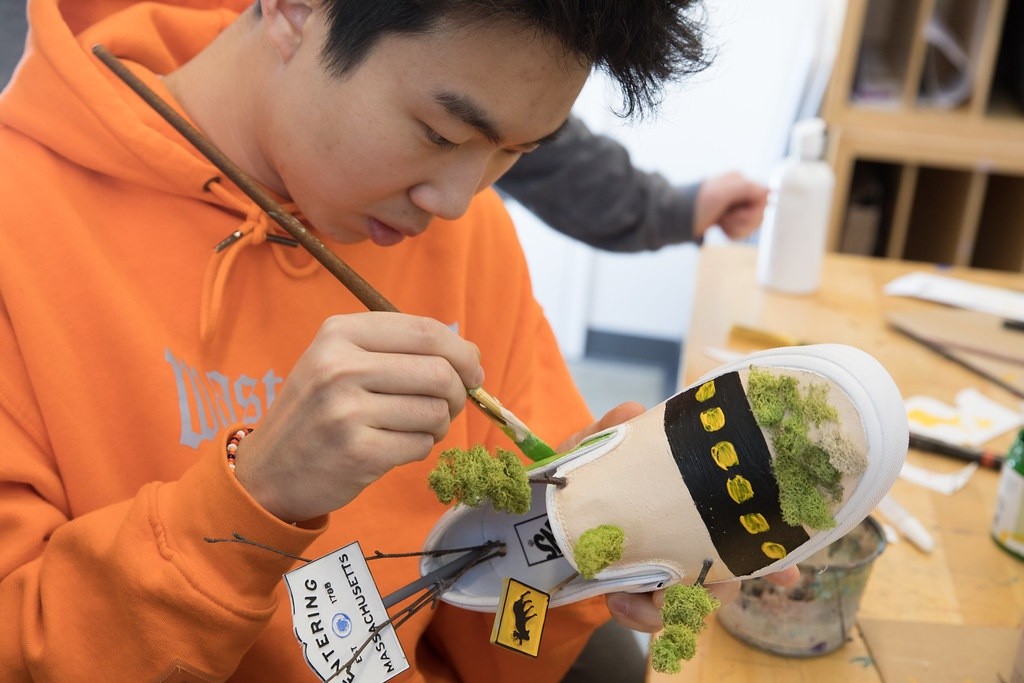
left=419, top=344, right=910, bottom=615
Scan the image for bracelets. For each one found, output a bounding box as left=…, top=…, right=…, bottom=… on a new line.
left=223, top=426, right=253, bottom=476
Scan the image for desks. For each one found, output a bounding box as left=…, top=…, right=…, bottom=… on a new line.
left=643, top=243, right=1024, bottom=683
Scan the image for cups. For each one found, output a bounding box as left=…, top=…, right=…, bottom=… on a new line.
left=716, top=517, right=887, bottom=659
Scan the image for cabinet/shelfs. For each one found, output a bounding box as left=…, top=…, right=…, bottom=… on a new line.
left=787, top=1, right=1024, bottom=272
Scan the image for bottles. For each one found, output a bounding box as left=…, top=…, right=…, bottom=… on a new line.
left=991, top=426, right=1024, bottom=561
left=760, top=118, right=835, bottom=294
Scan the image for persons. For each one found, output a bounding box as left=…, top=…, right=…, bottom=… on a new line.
left=490, top=110, right=772, bottom=253
left=1, top=1, right=799, bottom=683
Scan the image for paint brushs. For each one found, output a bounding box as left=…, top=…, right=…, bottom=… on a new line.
left=91, top=43, right=558, bottom=463
left=888, top=320, right=1024, bottom=398
